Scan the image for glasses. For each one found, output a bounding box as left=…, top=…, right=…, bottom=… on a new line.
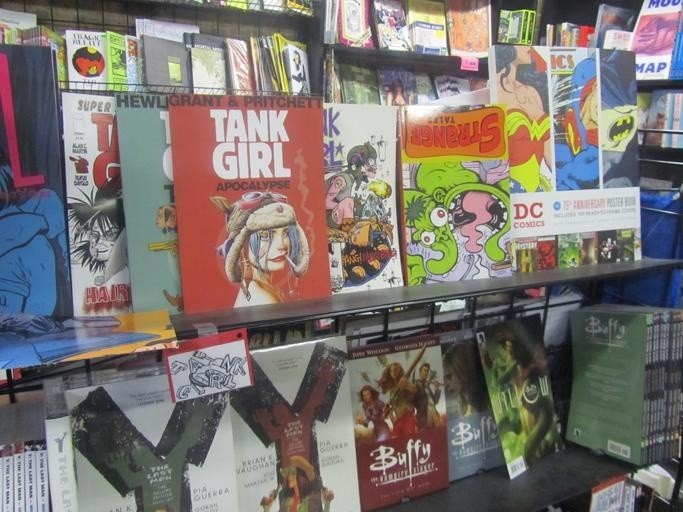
left=243, top=189, right=287, bottom=201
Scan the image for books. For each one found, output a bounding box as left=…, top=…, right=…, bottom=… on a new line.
left=59, top=93, right=332, bottom=318
left=0, top=330, right=361, bottom=512
left=650, top=88, right=683, bottom=149
left=629, top=0, right=683, bottom=80
left=491, top=42, right=642, bottom=273
left=538, top=2, right=628, bottom=41
left=335, top=64, right=515, bottom=295
left=347, top=298, right=683, bottom=512
left=1, top=6, right=313, bottom=96
left=325, top=0, right=494, bottom=63
left=496, top=10, right=535, bottom=45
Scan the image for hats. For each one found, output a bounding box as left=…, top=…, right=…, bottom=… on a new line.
left=214, top=190, right=313, bottom=284
